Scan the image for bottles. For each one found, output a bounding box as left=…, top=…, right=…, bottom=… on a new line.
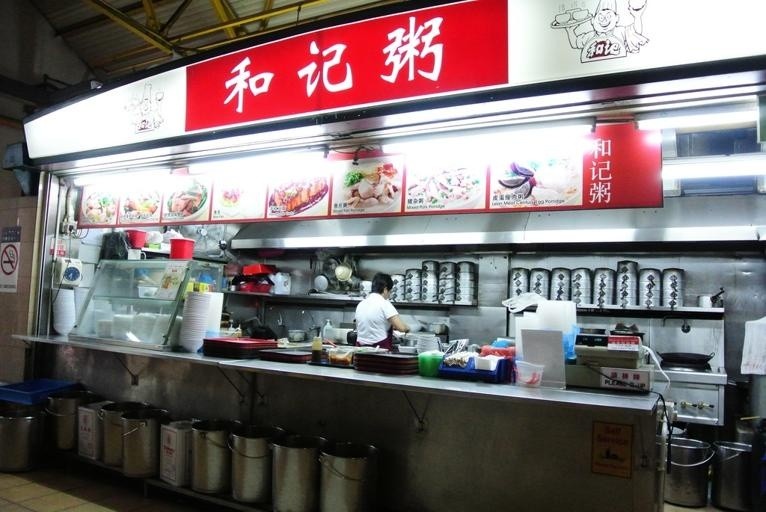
left=310, top=337, right=322, bottom=364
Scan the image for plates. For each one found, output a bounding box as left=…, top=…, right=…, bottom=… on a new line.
left=344, top=193, right=400, bottom=212
left=164, top=182, right=211, bottom=221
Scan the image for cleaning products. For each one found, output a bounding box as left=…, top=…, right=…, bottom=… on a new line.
left=324, top=318, right=333, bottom=344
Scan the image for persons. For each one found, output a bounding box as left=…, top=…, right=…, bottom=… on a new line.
left=355, top=272, right=410, bottom=351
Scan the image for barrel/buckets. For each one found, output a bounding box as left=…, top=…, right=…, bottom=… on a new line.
left=711, top=441, right=753, bottom=511
left=1, top=388, right=379, bottom=511
left=664, top=436, right=714, bottom=509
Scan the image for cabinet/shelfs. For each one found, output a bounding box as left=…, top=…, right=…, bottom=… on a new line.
left=68, top=259, right=228, bottom=351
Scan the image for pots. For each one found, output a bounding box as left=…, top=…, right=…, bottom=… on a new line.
left=654, top=350, right=715, bottom=363
left=348, top=260, right=475, bottom=305
left=509, top=260, right=725, bottom=307
left=418, top=319, right=446, bottom=334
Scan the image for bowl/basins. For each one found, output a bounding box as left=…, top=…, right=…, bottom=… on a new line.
left=398, top=334, right=436, bottom=355
left=51, top=288, right=211, bottom=353
left=288, top=330, right=305, bottom=342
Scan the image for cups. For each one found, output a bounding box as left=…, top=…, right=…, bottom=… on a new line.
left=127, top=249, right=147, bottom=261
